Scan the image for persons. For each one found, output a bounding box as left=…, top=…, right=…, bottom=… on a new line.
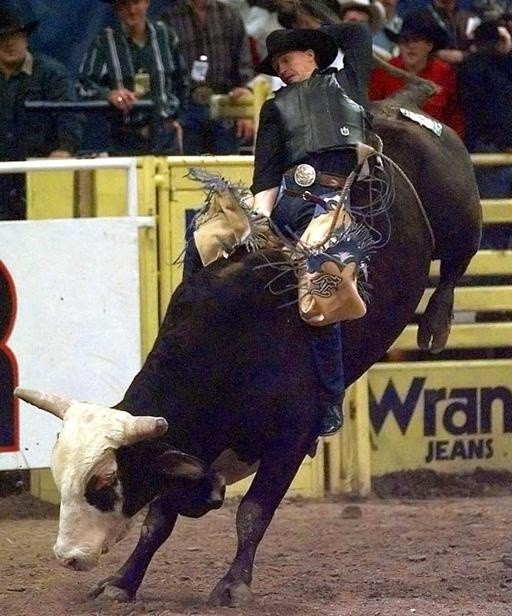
left=248, top=0, right=512, bottom=249
left=1, top=9, right=78, bottom=220
left=159, top=1, right=254, bottom=156
left=74, top=1, right=190, bottom=154
left=183, top=21, right=376, bottom=436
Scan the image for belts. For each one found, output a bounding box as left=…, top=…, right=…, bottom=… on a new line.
left=286, top=164, right=346, bottom=188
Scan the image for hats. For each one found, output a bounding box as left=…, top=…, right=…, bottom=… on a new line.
left=0, top=13, right=40, bottom=35
left=254, top=28, right=338, bottom=77
left=383, top=10, right=443, bottom=44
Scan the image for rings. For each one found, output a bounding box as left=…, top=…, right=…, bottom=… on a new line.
left=117, top=97, right=123, bottom=103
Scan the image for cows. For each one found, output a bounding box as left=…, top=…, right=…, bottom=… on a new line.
left=14, top=1, right=483, bottom=607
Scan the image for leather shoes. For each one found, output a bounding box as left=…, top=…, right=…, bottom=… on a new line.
left=319, top=399, right=344, bottom=437
